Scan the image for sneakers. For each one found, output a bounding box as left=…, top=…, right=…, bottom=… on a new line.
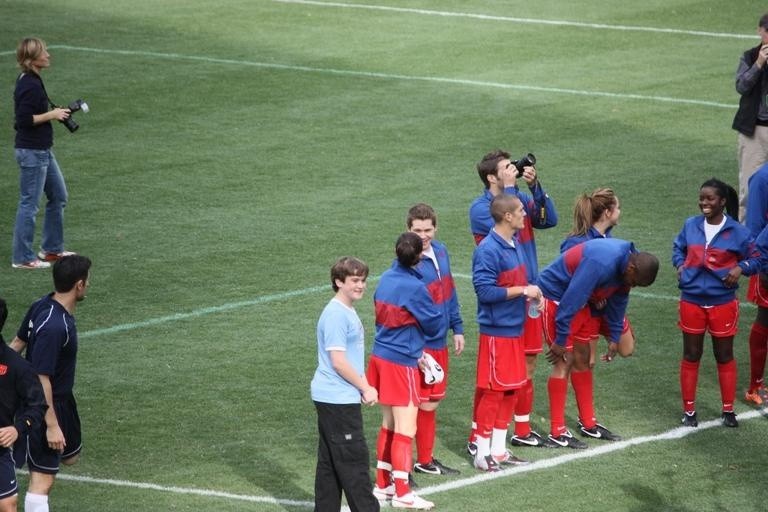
left=744, top=391, right=762, bottom=404
left=413, top=459, right=460, bottom=476
left=12, top=250, right=76, bottom=269
left=681, top=411, right=697, bottom=427
left=372, top=479, right=434, bottom=509
left=510, top=418, right=621, bottom=450
left=721, top=412, right=737, bottom=427
left=465, top=443, right=529, bottom=472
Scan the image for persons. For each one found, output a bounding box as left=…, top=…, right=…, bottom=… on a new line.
left=744, top=163, right=768, bottom=409
left=730, top=13, right=768, bottom=225
left=9, top=254, right=92, bottom=512
left=309, top=254, right=382, bottom=512
left=407, top=201, right=464, bottom=476
left=0, top=297, right=49, bottom=512
left=468, top=149, right=557, bottom=459
left=366, top=231, right=442, bottom=509
left=559, top=186, right=636, bottom=365
left=10, top=37, right=77, bottom=269
left=536, top=238, right=658, bottom=449
left=472, top=194, right=545, bottom=472
left=671, top=176, right=768, bottom=428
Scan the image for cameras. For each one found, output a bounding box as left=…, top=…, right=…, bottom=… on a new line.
left=506, top=153, right=536, bottom=178
left=51, top=97, right=90, bottom=133
